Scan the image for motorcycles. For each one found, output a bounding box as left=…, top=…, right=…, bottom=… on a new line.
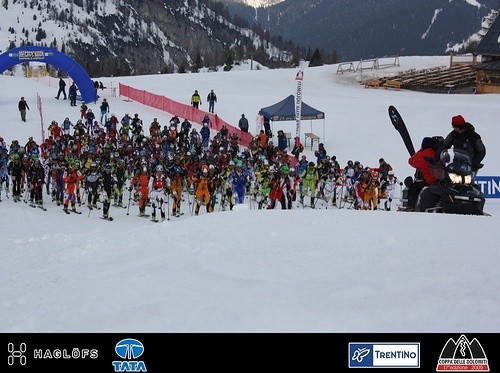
left=397, top=148, right=489, bottom=216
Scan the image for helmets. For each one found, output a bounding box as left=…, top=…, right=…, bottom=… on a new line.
left=229, top=161, right=235, bottom=166
left=156, top=165, right=163, bottom=172
left=209, top=165, right=215, bottom=170
left=203, top=168, right=208, bottom=173
left=280, top=165, right=289, bottom=174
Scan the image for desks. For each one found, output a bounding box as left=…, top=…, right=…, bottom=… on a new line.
left=285, top=132, right=292, bottom=150
left=304, top=132, right=320, bottom=151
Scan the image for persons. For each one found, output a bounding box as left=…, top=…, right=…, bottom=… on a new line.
left=0, top=116, right=396, bottom=220
left=53, top=75, right=67, bottom=99
left=67, top=81, right=79, bottom=106
left=206, top=89, right=218, bottom=114
left=81, top=101, right=87, bottom=120
left=439, top=114, right=489, bottom=180
left=96, top=96, right=114, bottom=124
left=189, top=87, right=202, bottom=112
left=16, top=93, right=30, bottom=124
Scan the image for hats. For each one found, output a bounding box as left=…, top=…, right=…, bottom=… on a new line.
left=452, top=115, right=465, bottom=128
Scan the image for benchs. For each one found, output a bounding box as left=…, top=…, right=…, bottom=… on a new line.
left=366, top=79, right=380, bottom=89
left=384, top=80, right=401, bottom=91
